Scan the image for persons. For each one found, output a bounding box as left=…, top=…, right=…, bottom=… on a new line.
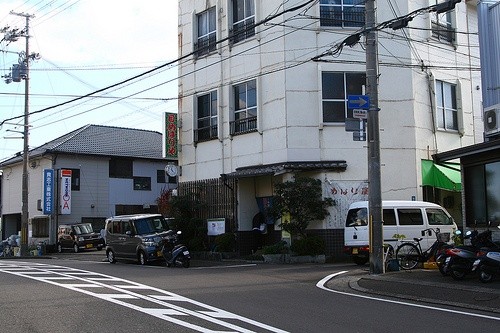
left=251, top=211, right=265, bottom=249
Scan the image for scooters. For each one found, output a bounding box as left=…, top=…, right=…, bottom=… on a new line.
left=156, top=231, right=191, bottom=268
left=434, top=218, right=500, bottom=285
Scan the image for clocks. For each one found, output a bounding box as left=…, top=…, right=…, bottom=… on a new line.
left=165, top=165, right=177, bottom=177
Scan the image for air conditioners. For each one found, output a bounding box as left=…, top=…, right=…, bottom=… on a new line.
left=484, top=103, right=500, bottom=137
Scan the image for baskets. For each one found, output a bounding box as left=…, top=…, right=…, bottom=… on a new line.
left=437, top=233, right=449, bottom=242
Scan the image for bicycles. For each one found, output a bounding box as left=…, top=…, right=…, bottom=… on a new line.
left=396, top=227, right=443, bottom=270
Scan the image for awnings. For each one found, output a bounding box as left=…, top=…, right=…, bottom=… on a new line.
left=433, top=163, right=462, bottom=192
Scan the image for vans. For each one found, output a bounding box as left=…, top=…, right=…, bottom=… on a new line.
left=105, top=214, right=177, bottom=265
left=57, top=222, right=104, bottom=253
left=343, top=200, right=459, bottom=264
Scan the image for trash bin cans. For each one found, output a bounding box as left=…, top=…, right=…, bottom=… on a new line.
left=386, top=258, right=402, bottom=272
